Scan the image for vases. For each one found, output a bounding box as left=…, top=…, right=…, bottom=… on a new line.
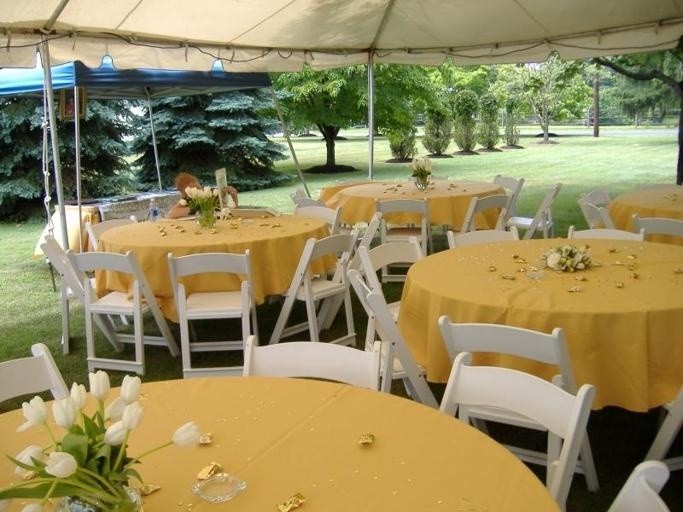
left=66, top=486, right=142, bottom=512
left=195, top=212, right=216, bottom=233
left=414, top=175, right=429, bottom=191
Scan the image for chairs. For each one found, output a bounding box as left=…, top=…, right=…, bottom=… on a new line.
left=492, top=174, right=524, bottom=221
left=242, top=334, right=381, bottom=392
left=358, top=236, right=424, bottom=325
left=322, top=211, right=383, bottom=331
left=568, top=225, right=644, bottom=242
left=506, top=183, right=563, bottom=241
left=85, top=216, right=139, bottom=325
left=577, top=187, right=611, bottom=229
left=374, top=198, right=432, bottom=282
left=290, top=188, right=362, bottom=253
left=346, top=269, right=439, bottom=410
left=448, top=226, right=520, bottom=250
left=608, top=460, right=671, bottom=512
left=641, top=384, right=683, bottom=472
left=166, top=250, right=256, bottom=380
left=633, top=215, right=683, bottom=237
left=408, top=175, right=448, bottom=236
left=270, top=229, right=361, bottom=347
left=438, top=351, right=596, bottom=511
left=438, top=317, right=598, bottom=492
left=270, top=203, right=343, bottom=311
left=462, top=193, right=515, bottom=233
left=1, top=344, right=70, bottom=403
left=41, top=236, right=116, bottom=355
left=64, top=249, right=179, bottom=376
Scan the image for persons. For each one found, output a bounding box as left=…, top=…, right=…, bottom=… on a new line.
left=168, top=172, right=238, bottom=218
left=64, top=96, right=75, bottom=116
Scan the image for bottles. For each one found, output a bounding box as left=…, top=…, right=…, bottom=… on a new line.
left=149, top=198, right=159, bottom=222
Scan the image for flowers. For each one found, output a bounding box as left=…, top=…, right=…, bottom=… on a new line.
left=540, top=242, right=593, bottom=273
left=1, top=371, right=200, bottom=511
left=178, top=186, right=221, bottom=228
left=410, top=155, right=432, bottom=186
